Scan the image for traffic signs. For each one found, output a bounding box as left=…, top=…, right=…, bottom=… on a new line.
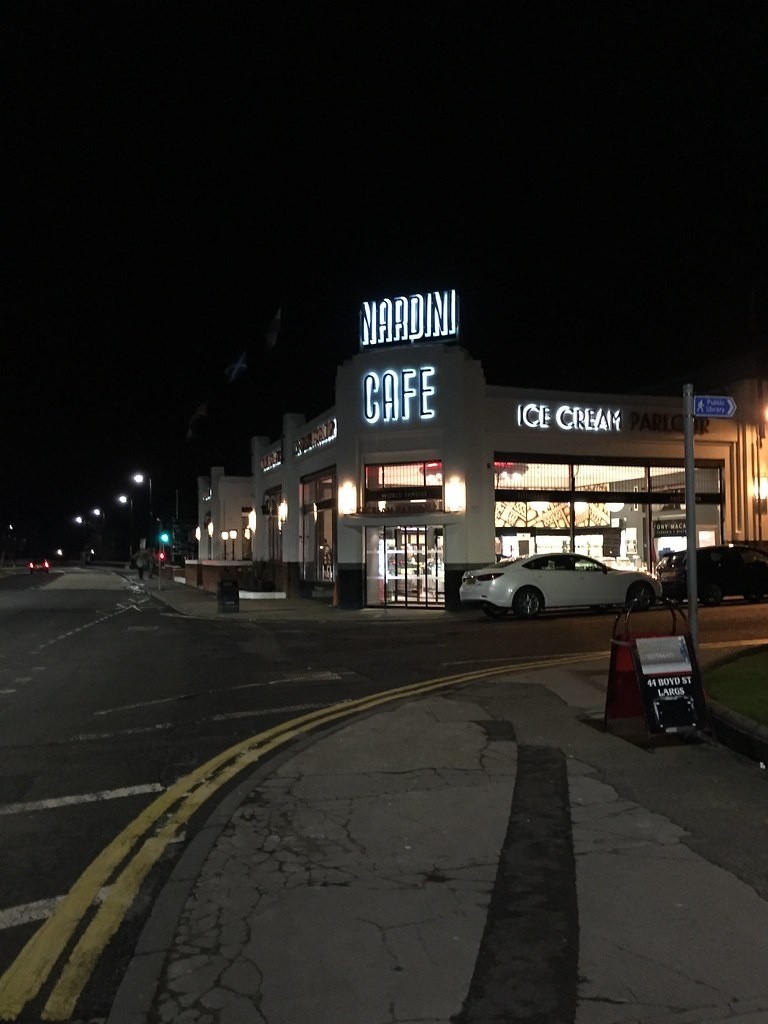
left=692, top=395, right=737, bottom=417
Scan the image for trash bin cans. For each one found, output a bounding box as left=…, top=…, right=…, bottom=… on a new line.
left=216, top=578, right=239, bottom=611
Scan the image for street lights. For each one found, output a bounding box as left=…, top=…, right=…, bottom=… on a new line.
left=119, top=495, right=133, bottom=560
left=133, top=474, right=152, bottom=579
left=93, top=507, right=106, bottom=565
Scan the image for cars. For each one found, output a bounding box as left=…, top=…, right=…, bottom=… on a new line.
left=457, top=552, right=664, bottom=620
left=656, top=544, right=768, bottom=607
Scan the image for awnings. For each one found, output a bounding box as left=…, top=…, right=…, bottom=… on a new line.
left=345, top=511, right=459, bottom=527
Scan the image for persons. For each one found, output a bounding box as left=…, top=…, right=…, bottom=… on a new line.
left=137, top=556, right=146, bottom=580
left=148, top=556, right=154, bottom=578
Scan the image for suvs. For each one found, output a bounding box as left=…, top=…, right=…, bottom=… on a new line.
left=29, top=558, right=50, bottom=574
